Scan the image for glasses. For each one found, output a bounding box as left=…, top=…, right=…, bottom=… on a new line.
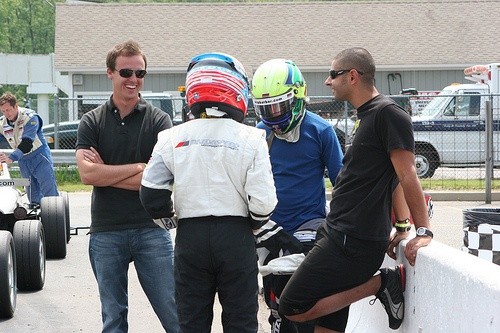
left=328, top=69, right=364, bottom=80
left=112, top=68, right=147, bottom=78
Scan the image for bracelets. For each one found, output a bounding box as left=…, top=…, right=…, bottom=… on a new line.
left=395, top=218, right=411, bottom=232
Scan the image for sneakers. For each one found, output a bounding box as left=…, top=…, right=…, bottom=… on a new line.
left=369, top=264, right=406, bottom=330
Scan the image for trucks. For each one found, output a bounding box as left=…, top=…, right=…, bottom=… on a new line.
left=323, top=96, right=434, bottom=152
left=408, top=64, right=500, bottom=179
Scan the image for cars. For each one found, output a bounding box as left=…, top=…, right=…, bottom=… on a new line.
left=42, top=96, right=188, bottom=151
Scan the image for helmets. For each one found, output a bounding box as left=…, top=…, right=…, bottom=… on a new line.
left=252, top=58, right=307, bottom=133
left=187, top=53, right=250, bottom=124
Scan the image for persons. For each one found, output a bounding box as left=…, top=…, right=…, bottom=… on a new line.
left=0, top=92, right=59, bottom=207
left=139, top=53, right=278, bottom=333
left=74, top=41, right=182, bottom=333
left=249, top=59, right=346, bottom=333
left=280, top=46, right=433, bottom=333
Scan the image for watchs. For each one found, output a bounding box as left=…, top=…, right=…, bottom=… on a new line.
left=416, top=228, right=434, bottom=239
